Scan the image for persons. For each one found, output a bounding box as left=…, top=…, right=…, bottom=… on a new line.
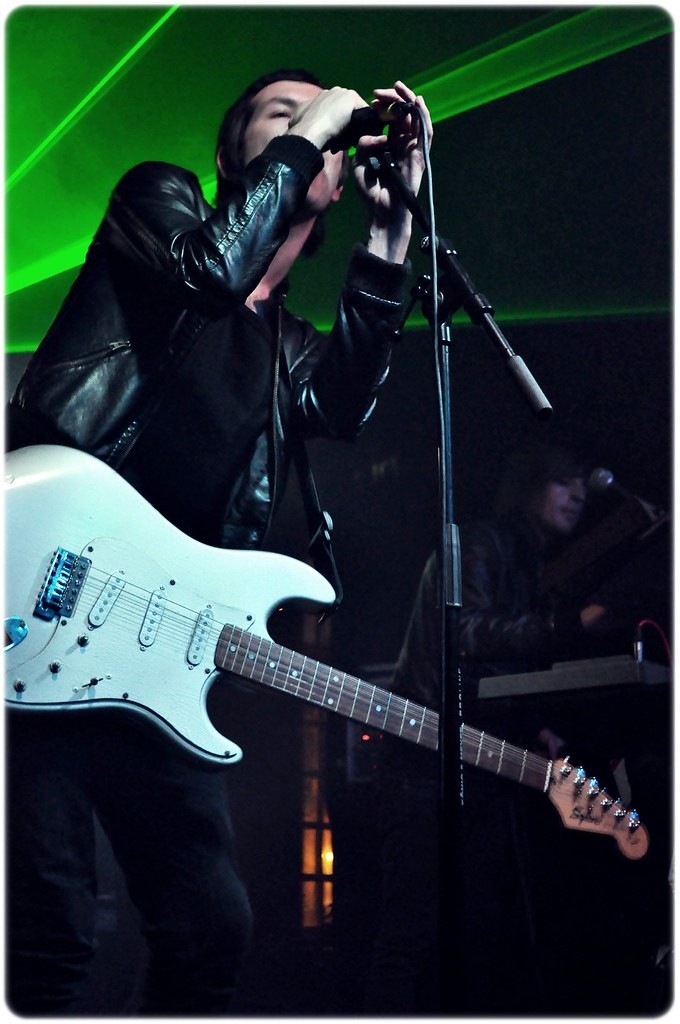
left=367, top=432, right=608, bottom=1015
left=6, top=69, right=431, bottom=1017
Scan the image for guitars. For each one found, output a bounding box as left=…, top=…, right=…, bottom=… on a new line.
left=2, top=438, right=652, bottom=865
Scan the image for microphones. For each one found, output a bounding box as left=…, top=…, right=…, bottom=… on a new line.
left=320, top=102, right=416, bottom=154
left=588, top=467, right=666, bottom=521
